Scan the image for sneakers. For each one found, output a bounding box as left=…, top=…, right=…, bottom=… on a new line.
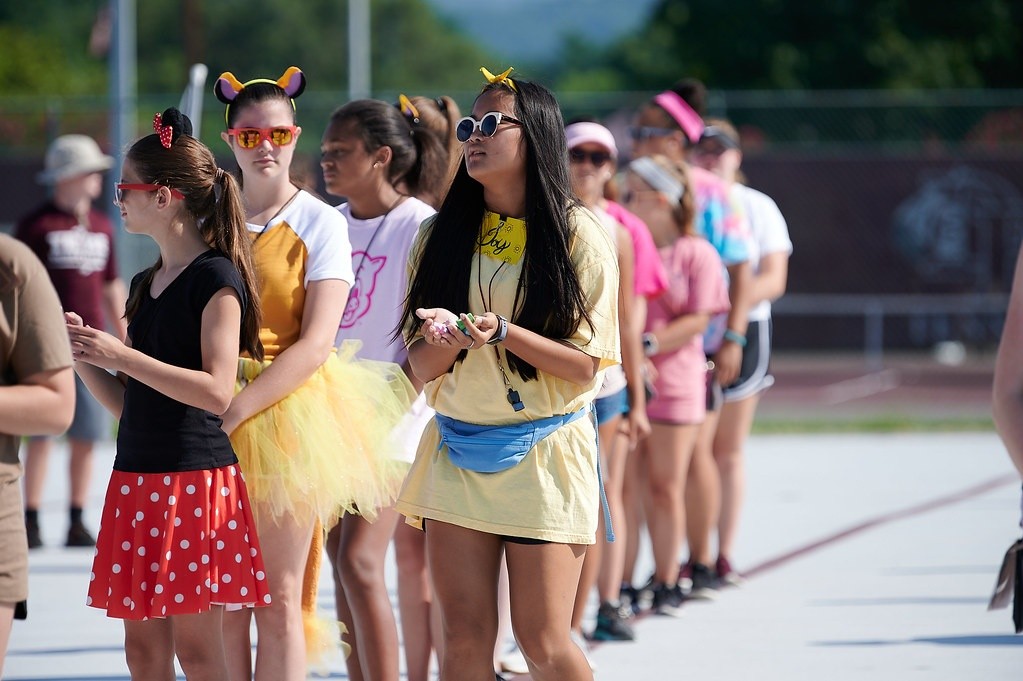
left=593, top=556, right=744, bottom=641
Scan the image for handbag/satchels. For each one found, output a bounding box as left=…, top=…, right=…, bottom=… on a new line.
left=434, top=403, right=593, bottom=473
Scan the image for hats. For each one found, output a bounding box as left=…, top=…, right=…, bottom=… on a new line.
left=35, top=134, right=115, bottom=186
left=564, top=122, right=620, bottom=160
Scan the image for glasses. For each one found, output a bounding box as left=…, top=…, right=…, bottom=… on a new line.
left=627, top=127, right=672, bottom=140
left=114, top=181, right=186, bottom=202
left=230, top=125, right=299, bottom=150
left=566, top=147, right=613, bottom=167
left=693, top=145, right=728, bottom=157
left=455, top=111, right=524, bottom=143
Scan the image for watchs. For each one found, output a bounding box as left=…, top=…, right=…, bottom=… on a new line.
left=486, top=314, right=507, bottom=345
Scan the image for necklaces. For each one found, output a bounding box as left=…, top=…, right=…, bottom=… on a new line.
left=355, top=194, right=403, bottom=281
left=251, top=190, right=301, bottom=247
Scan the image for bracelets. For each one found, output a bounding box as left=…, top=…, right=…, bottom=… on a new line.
left=74, top=351, right=84, bottom=362
left=643, top=332, right=660, bottom=357
left=723, top=328, right=747, bottom=346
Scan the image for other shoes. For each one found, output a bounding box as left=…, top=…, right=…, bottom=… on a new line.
left=65, top=526, right=96, bottom=546
left=571, top=630, right=595, bottom=670
left=500, top=644, right=530, bottom=674
left=27, top=527, right=42, bottom=548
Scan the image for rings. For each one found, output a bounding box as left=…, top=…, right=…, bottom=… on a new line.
left=468, top=339, right=475, bottom=347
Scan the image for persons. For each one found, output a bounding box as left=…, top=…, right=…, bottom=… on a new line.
left=12, top=133, right=128, bottom=548
left=392, top=78, right=622, bottom=681
left=393, top=94, right=464, bottom=681
left=993, top=240, right=1022, bottom=526
left=500, top=79, right=794, bottom=674
left=66, top=109, right=272, bottom=681
left=0, top=232, right=76, bottom=681
left=322, top=99, right=439, bottom=681
left=222, top=78, right=355, bottom=681
